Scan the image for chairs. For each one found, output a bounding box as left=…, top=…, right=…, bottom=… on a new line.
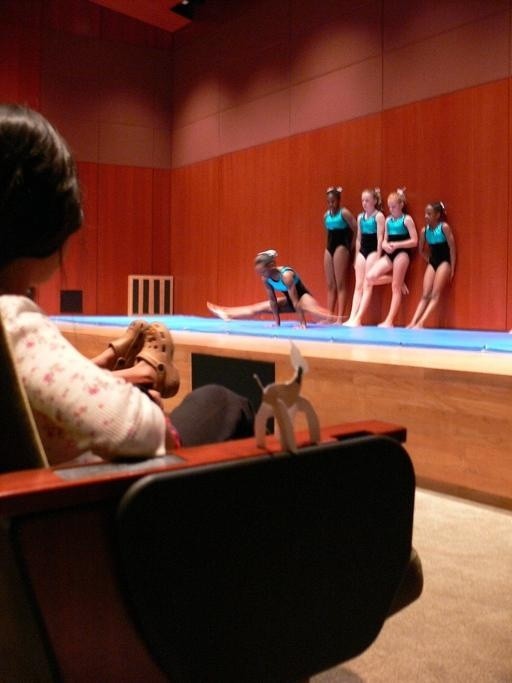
left=0, top=313, right=424, bottom=682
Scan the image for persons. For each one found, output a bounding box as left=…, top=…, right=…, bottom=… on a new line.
left=343, top=188, right=384, bottom=327
left=320, top=186, right=356, bottom=326
left=366, top=185, right=419, bottom=328
left=93, top=319, right=181, bottom=398
left=0, top=104, right=265, bottom=465
left=207, top=249, right=347, bottom=321
left=405, top=201, right=458, bottom=328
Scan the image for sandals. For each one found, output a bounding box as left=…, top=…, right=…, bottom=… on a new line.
left=108, top=320, right=180, bottom=399
